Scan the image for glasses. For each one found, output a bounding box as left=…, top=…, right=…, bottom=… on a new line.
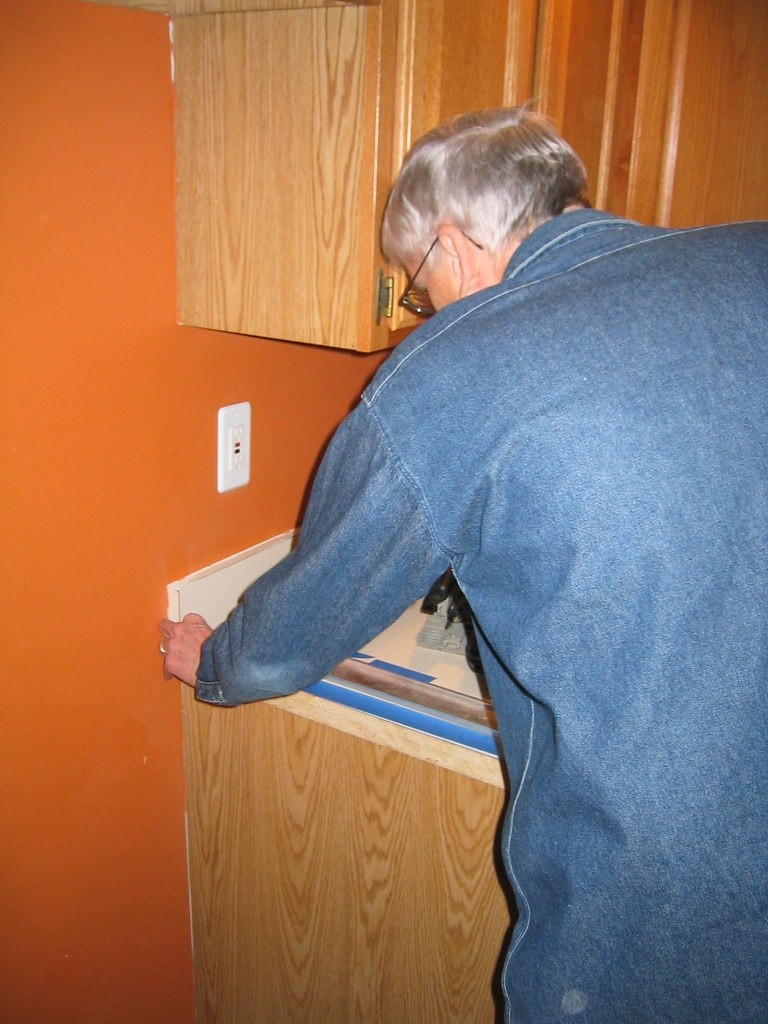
left=398, top=232, right=483, bottom=319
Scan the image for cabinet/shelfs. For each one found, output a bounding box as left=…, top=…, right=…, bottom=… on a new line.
left=172, top=0, right=768, bottom=1024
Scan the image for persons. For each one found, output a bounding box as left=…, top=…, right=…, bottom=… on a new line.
left=160, top=97, right=768, bottom=1024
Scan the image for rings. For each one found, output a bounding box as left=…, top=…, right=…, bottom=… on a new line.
left=159, top=638, right=168, bottom=654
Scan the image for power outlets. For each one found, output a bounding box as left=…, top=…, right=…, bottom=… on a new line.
left=216, top=400, right=253, bottom=493
